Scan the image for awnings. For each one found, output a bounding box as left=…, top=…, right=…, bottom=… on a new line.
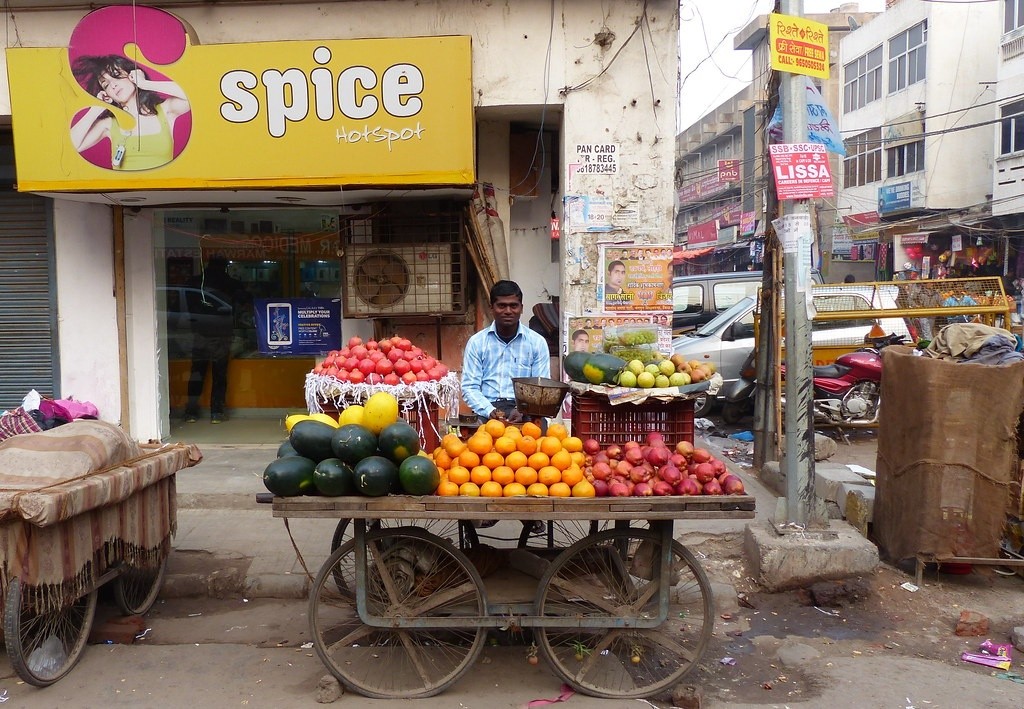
left=901, top=231, right=945, bottom=244
left=674, top=242, right=749, bottom=259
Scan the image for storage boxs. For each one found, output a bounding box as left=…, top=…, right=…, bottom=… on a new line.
left=602, top=323, right=659, bottom=362
left=166, top=219, right=340, bottom=285
left=571, top=391, right=698, bottom=454
left=316, top=387, right=441, bottom=454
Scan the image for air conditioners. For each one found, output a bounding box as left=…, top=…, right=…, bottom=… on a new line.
left=345, top=244, right=454, bottom=314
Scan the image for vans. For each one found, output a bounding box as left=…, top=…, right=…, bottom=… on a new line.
left=671, top=268, right=825, bottom=341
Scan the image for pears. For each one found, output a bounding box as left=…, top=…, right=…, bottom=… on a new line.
left=619, top=353, right=716, bottom=387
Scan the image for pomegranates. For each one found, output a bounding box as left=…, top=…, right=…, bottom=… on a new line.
left=312, top=334, right=448, bottom=385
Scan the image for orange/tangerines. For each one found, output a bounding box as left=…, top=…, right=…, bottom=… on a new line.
left=427, top=419, right=596, bottom=497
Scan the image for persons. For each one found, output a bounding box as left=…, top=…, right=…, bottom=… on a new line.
left=747, top=263, right=753, bottom=272
left=183, top=253, right=247, bottom=423
left=570, top=315, right=672, bottom=352
left=943, top=282, right=978, bottom=326
left=71, top=55, right=191, bottom=172
left=605, top=248, right=674, bottom=307
left=1002, top=268, right=1016, bottom=296
left=840, top=274, right=856, bottom=284
left=461, top=280, right=550, bottom=534
left=908, top=278, right=945, bottom=343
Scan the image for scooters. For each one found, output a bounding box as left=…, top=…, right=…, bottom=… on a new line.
left=723, top=344, right=788, bottom=426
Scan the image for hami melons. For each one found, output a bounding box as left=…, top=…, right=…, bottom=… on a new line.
left=284, top=392, right=399, bottom=436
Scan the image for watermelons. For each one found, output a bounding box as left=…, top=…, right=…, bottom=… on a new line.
left=563, top=350, right=662, bottom=386
left=262, top=417, right=440, bottom=497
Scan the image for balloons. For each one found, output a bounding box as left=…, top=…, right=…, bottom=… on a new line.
left=906, top=244, right=924, bottom=259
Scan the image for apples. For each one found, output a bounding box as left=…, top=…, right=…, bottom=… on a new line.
left=583, top=432, right=745, bottom=497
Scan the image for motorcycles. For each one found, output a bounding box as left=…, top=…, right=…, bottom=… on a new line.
left=748, top=333, right=912, bottom=446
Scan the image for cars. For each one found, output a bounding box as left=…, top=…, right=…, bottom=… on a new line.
left=669, top=284, right=922, bottom=420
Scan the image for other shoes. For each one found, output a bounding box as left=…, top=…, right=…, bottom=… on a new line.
left=475, top=520, right=499, bottom=528
left=520, top=520, right=546, bottom=533
left=211, top=413, right=229, bottom=423
left=186, top=411, right=197, bottom=423
